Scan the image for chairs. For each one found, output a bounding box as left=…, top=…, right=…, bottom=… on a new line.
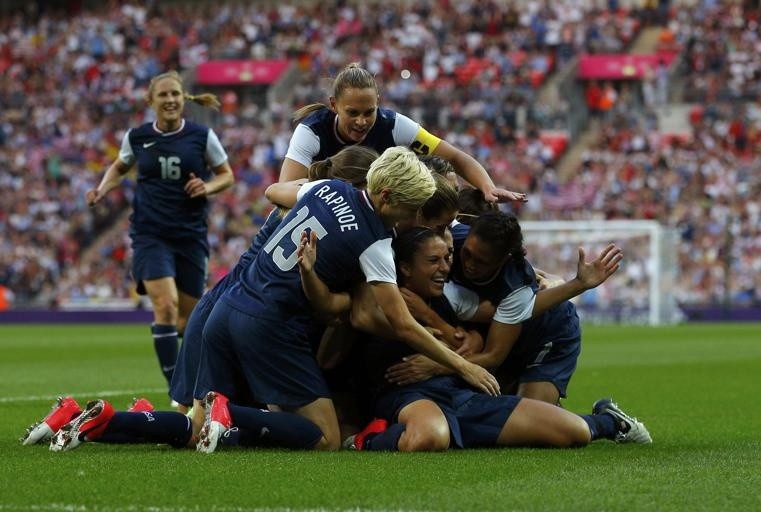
left=196, top=391, right=233, bottom=453
left=592, top=399, right=652, bottom=443
left=342, top=418, right=388, bottom=450
left=17, top=395, right=155, bottom=452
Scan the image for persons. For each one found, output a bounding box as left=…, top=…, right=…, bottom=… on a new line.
left=19, top=147, right=378, bottom=454
left=0, top=1, right=381, bottom=301
left=369, top=1, right=761, bottom=307
left=195, top=65, right=653, bottom=453
left=86, top=74, right=233, bottom=397
